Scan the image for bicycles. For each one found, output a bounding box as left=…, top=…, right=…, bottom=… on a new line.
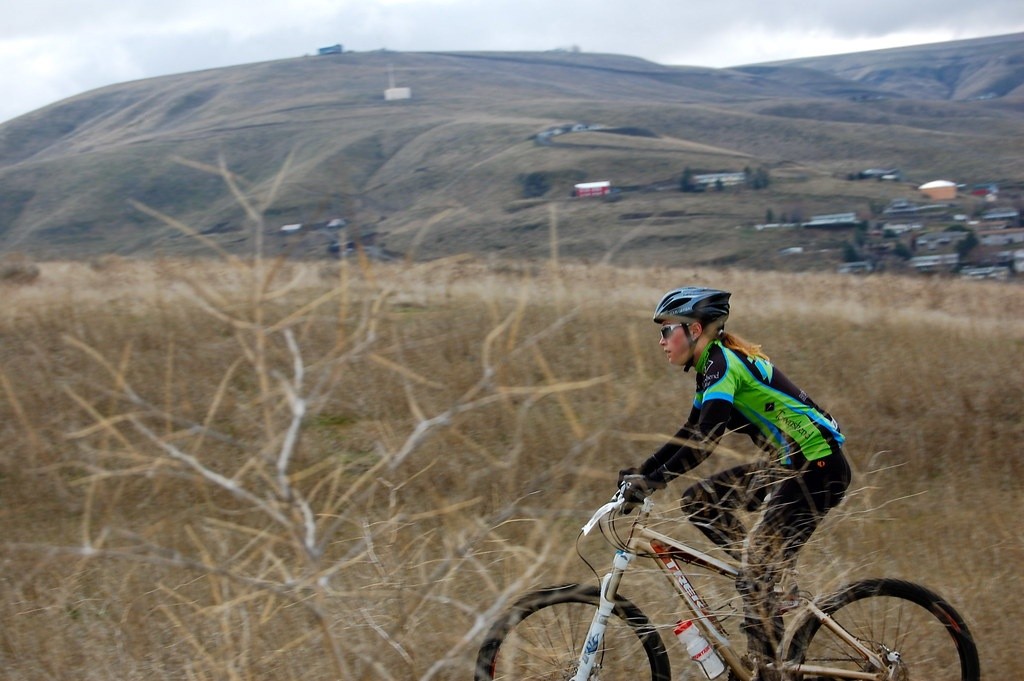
left=474, top=475, right=980, bottom=681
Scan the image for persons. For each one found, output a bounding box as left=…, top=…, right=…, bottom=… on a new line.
left=617, top=286, right=853, bottom=681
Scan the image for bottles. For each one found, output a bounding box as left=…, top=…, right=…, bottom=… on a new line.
left=673, top=619, right=725, bottom=679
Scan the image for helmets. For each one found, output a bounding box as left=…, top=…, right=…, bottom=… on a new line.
left=652, top=286, right=731, bottom=324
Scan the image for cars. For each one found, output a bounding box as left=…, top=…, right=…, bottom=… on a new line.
left=326, top=218, right=347, bottom=228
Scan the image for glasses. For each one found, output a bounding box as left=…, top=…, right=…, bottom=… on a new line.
left=661, top=324, right=690, bottom=340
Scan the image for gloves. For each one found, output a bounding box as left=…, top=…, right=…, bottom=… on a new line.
left=617, top=468, right=640, bottom=488
left=621, top=475, right=647, bottom=514
